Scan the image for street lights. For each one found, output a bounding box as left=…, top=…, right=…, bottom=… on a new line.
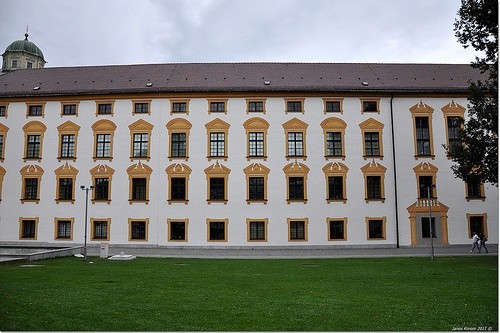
left=80, top=185, right=96, bottom=261
left=421, top=183, right=435, bottom=261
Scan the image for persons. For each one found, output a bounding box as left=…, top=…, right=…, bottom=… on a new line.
left=478, top=231, right=488, bottom=254
left=470, top=232, right=480, bottom=254
